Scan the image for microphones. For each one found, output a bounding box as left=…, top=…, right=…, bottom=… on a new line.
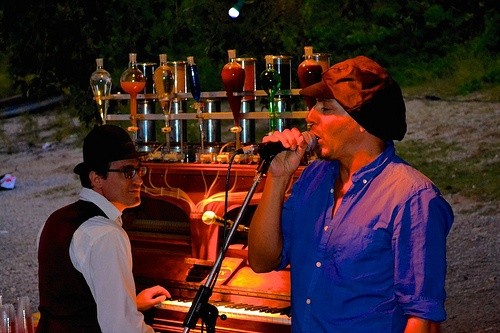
left=201, top=210, right=250, bottom=233
left=236, top=131, right=315, bottom=154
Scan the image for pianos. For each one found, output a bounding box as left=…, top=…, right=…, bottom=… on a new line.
left=120, top=162, right=311, bottom=333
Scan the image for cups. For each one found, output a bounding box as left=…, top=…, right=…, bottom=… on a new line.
left=16, top=296, right=34, bottom=333
left=0, top=296, right=16, bottom=333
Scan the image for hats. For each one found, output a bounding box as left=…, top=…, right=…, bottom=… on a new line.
left=83, top=124, right=149, bottom=163
left=299, top=55, right=407, bottom=142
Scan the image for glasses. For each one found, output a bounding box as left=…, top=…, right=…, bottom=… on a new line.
left=96, top=166, right=147, bottom=179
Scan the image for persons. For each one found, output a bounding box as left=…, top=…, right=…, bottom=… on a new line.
left=35, top=124, right=172, bottom=333
left=247, top=55, right=454, bottom=333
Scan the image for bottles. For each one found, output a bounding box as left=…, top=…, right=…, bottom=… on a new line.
left=298, top=47, right=323, bottom=130
left=90, top=59, right=112, bottom=125
left=259, top=55, right=281, bottom=131
left=221, top=50, right=245, bottom=133
left=186, top=56, right=201, bottom=107
left=120, top=53, right=146, bottom=131
left=153, top=54, right=175, bottom=132
